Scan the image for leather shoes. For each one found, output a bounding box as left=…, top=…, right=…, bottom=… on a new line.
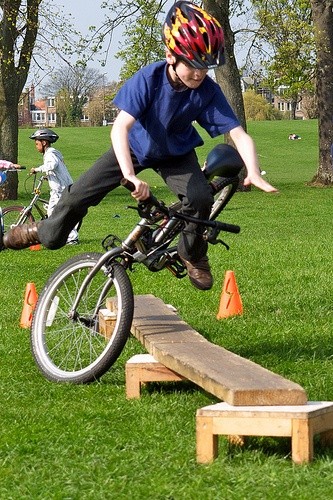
left=3, top=222, right=42, bottom=250
left=179, top=254, right=213, bottom=290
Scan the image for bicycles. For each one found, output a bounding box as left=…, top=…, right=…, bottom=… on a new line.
left=1, top=171, right=83, bottom=236
left=28, top=142, right=246, bottom=387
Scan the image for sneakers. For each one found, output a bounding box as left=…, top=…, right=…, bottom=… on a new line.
left=67, top=240, right=80, bottom=246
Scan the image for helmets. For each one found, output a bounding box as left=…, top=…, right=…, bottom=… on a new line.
left=28, top=129, right=59, bottom=143
left=161, top=1, right=226, bottom=69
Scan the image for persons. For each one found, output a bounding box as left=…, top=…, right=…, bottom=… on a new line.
left=289, top=133, right=302, bottom=140
left=3, top=0, right=278, bottom=291
left=29, top=128, right=79, bottom=245
left=0, top=159, right=21, bottom=171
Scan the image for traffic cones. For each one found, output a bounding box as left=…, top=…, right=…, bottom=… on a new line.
left=19, top=282, right=39, bottom=330
left=215, top=269, right=244, bottom=321
